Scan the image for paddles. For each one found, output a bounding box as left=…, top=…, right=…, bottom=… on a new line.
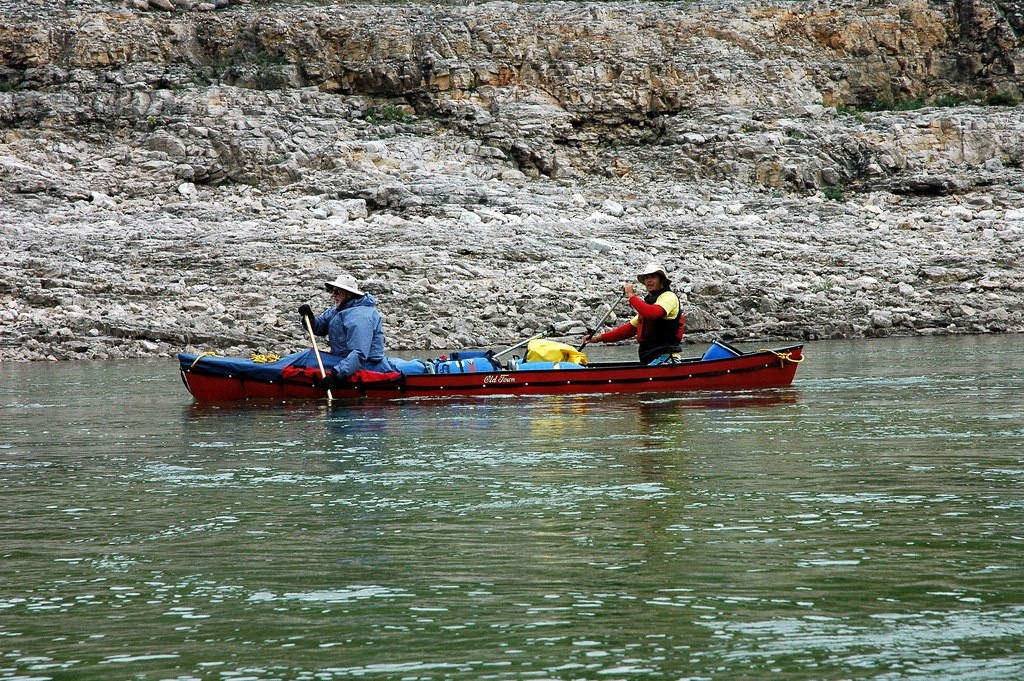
left=577, top=286, right=625, bottom=353
left=492, top=325, right=556, bottom=358
left=304, top=314, right=334, bottom=400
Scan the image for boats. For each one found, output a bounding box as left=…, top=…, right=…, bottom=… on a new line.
left=179, top=342, right=803, bottom=400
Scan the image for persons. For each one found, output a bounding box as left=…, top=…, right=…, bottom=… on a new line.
left=582, top=263, right=683, bottom=369
left=297, top=274, right=386, bottom=392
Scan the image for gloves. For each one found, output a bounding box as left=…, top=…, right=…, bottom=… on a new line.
left=318, top=369, right=337, bottom=389
left=299, top=304, right=314, bottom=320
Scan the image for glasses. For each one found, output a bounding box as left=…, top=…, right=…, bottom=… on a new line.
left=329, top=288, right=342, bottom=295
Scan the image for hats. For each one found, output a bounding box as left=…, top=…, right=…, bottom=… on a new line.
left=325, top=275, right=364, bottom=296
left=637, top=264, right=672, bottom=286
